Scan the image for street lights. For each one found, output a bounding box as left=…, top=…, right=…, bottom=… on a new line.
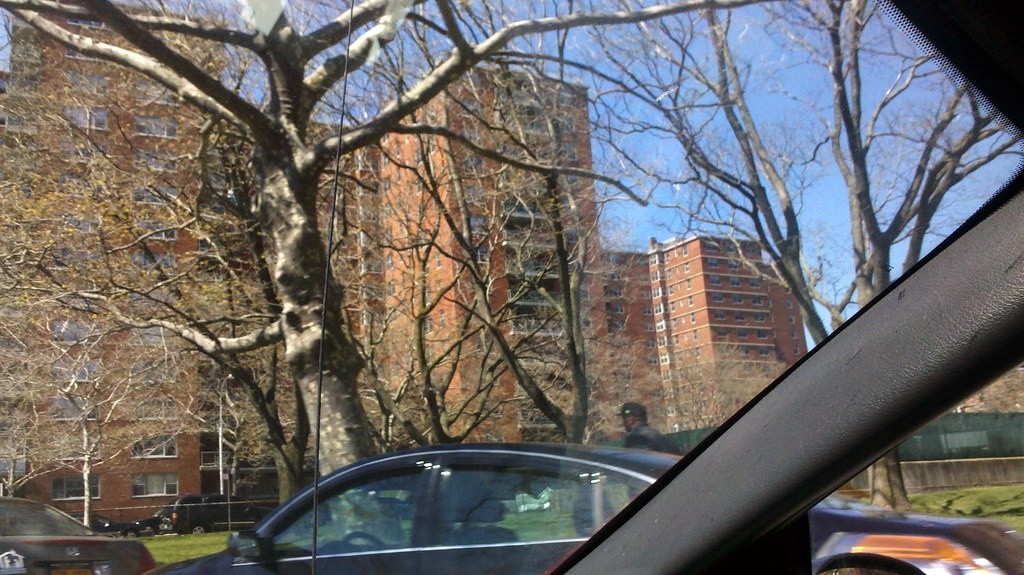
left=219, top=373, right=236, bottom=496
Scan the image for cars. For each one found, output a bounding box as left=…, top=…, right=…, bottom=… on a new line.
left=0, top=494, right=158, bottom=574
left=66, top=509, right=140, bottom=538
left=145, top=437, right=1024, bottom=574
left=158, top=490, right=274, bottom=535
left=134, top=495, right=180, bottom=534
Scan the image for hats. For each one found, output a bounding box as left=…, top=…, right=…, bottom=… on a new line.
left=616, top=403, right=647, bottom=415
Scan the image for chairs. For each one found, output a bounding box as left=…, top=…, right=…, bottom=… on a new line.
left=345, top=499, right=405, bottom=544
left=442, top=499, right=517, bottom=542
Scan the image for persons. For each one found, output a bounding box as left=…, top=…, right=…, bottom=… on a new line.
left=604, top=402, right=686, bottom=501
